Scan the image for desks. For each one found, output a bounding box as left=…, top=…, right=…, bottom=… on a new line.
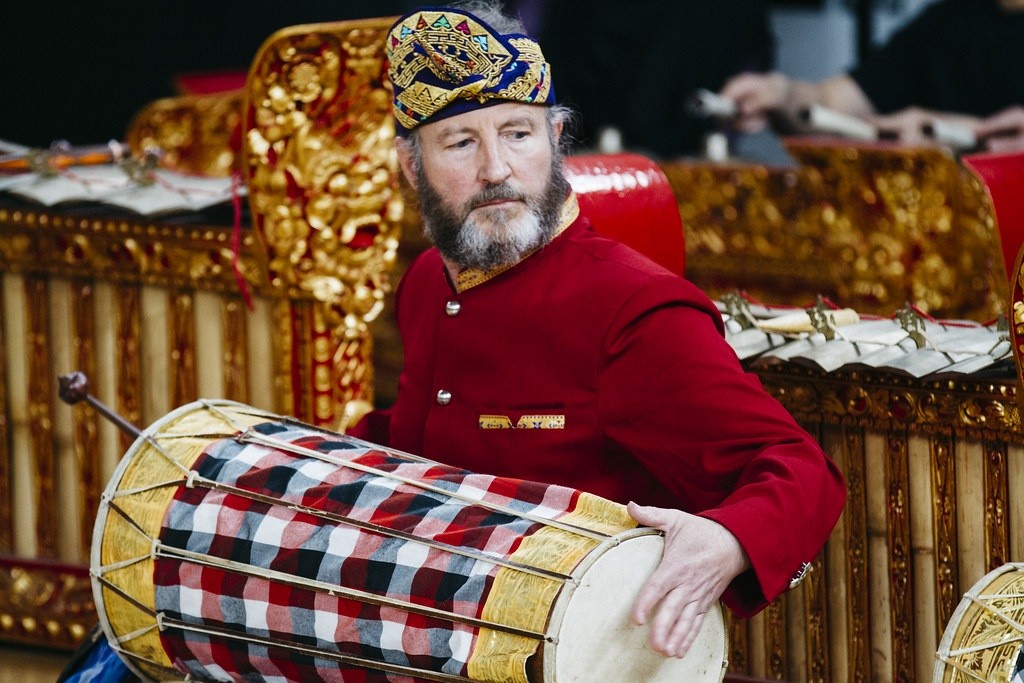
left=721, top=334, right=1024, bottom=683
left=1, top=196, right=337, bottom=653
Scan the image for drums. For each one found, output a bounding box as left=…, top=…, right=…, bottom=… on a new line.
left=92, top=399, right=727, bottom=683
left=933, top=559, right=1024, bottom=683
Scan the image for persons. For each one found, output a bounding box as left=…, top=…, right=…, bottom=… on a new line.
left=56, top=0, right=847, bottom=683
left=722, top=1, right=1024, bottom=149
